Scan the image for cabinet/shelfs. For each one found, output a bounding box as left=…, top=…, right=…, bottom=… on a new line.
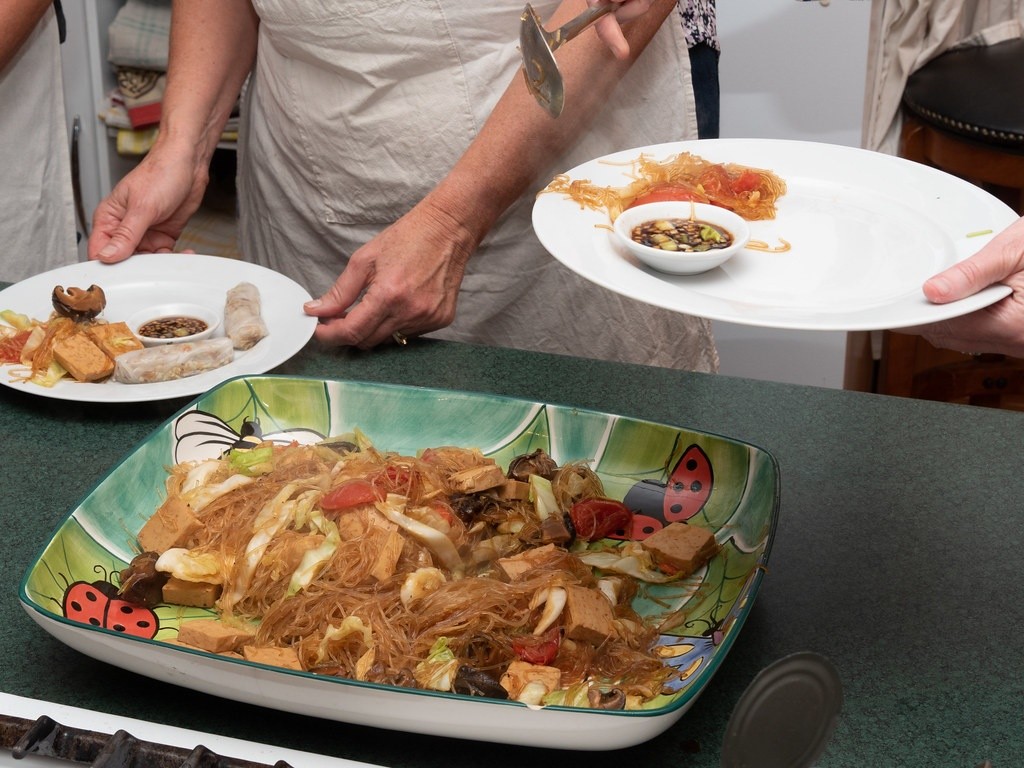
left=57, top=0, right=237, bottom=262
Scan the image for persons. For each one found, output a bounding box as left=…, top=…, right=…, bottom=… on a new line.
left=87, top=0, right=719, bottom=375
left=0, top=0, right=81, bottom=282
left=587, top=0, right=1024, bottom=362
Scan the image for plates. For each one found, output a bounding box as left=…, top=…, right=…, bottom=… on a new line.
left=532, top=138, right=1023, bottom=331
left=17, top=376, right=780, bottom=753
left=1, top=254, right=320, bottom=404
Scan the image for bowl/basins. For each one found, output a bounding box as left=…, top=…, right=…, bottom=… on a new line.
left=125, top=302, right=220, bottom=345
left=611, top=200, right=750, bottom=273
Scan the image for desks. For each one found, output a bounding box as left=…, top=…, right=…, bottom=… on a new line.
left=1, top=337, right=1024, bottom=768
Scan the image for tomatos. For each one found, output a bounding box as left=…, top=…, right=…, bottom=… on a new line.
left=626, top=164, right=765, bottom=213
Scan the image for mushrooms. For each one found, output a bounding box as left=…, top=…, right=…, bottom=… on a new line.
left=51, top=284, right=106, bottom=319
left=120, top=440, right=625, bottom=713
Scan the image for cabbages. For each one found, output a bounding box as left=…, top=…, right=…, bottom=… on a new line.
left=153, top=428, right=683, bottom=712
left=0, top=309, right=68, bottom=386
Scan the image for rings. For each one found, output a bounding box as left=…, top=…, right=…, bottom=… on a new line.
left=391, top=332, right=408, bottom=347
left=961, top=351, right=981, bottom=359
